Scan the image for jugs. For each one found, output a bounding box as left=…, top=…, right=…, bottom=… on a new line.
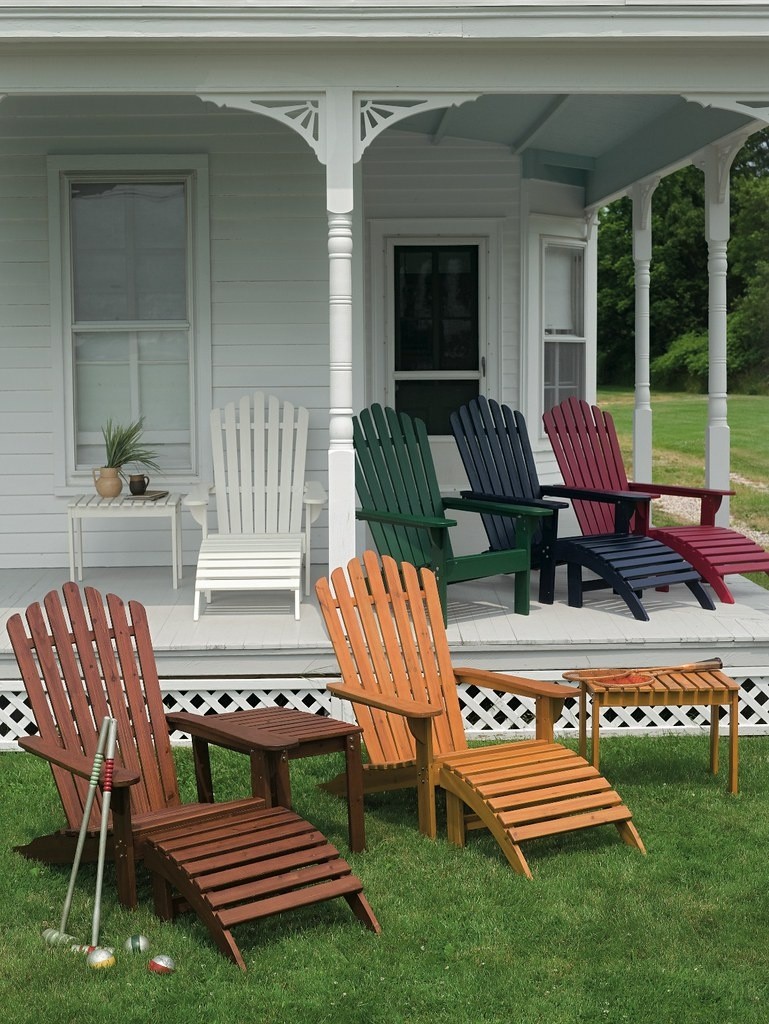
left=129, top=474, right=149, bottom=495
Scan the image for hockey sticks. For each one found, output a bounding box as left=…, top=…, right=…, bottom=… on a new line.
left=69, top=718, right=117, bottom=957
left=39, top=715, right=110, bottom=950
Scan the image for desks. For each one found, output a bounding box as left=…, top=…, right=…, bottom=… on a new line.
left=191, top=708, right=367, bottom=854
left=579, top=669, right=741, bottom=795
left=66, top=494, right=183, bottom=590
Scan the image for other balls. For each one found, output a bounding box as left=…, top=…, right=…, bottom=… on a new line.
left=124, top=934, right=150, bottom=954
left=87, top=949, right=116, bottom=972
left=148, top=955, right=176, bottom=975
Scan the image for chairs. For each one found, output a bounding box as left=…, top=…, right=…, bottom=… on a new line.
left=7, top=582, right=380, bottom=972
left=451, top=395, right=716, bottom=622
left=314, top=550, right=647, bottom=881
left=542, top=397, right=769, bottom=604
left=182, top=391, right=329, bottom=621
left=352, top=403, right=553, bottom=628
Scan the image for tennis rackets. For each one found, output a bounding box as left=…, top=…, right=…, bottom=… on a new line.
left=561, top=657, right=723, bottom=688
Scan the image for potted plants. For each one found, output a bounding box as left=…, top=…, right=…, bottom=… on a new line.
left=92, top=415, right=170, bottom=497
